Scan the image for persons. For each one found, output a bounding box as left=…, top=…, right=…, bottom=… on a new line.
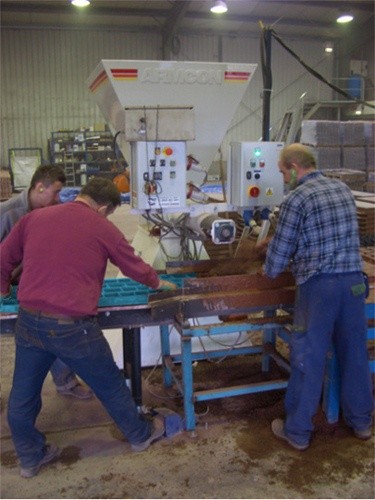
left=258, top=143, right=375, bottom=451
left=0, top=164, right=95, bottom=400
left=0, top=181, right=178, bottom=478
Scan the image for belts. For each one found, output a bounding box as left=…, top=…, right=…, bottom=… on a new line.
left=20, top=306, right=89, bottom=319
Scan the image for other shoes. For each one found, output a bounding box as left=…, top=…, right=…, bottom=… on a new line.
left=272, top=418, right=309, bottom=450
left=20, top=444, right=55, bottom=478
left=353, top=428, right=372, bottom=439
left=56, top=379, right=91, bottom=399
left=131, top=415, right=165, bottom=450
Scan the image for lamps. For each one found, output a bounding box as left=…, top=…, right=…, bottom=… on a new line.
left=336, top=6, right=354, bottom=24
left=209, top=0, right=228, bottom=13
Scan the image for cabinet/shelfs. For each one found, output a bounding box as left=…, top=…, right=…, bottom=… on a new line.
left=50, top=131, right=127, bottom=185
